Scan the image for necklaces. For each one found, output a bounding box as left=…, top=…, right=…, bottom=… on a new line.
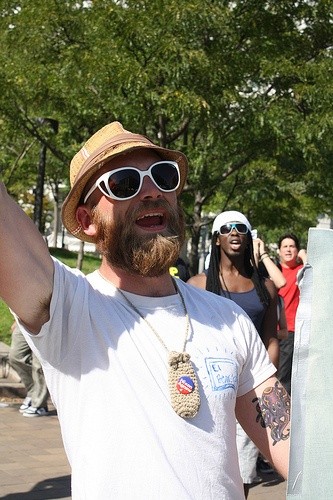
left=98, top=267, right=201, bottom=420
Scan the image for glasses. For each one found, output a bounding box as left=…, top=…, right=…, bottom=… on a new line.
left=84, top=161, right=180, bottom=204
left=216, top=223, right=248, bottom=236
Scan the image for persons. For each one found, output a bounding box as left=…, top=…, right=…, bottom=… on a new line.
left=274, top=231, right=307, bottom=397
left=8, top=319, right=51, bottom=417
left=185, top=210, right=280, bottom=500
left=252, top=237, right=288, bottom=289
left=1, top=121, right=293, bottom=500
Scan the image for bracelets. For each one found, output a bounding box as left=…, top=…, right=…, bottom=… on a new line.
left=260, top=251, right=268, bottom=256
left=261, top=256, right=269, bottom=263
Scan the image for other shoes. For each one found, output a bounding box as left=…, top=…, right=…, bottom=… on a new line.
left=257, top=454, right=275, bottom=473
left=252, top=476, right=262, bottom=484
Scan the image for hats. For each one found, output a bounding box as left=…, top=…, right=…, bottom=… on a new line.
left=212, top=211, right=251, bottom=236
left=60, top=122, right=188, bottom=243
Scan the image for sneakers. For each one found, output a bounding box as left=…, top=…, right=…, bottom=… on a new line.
left=23, top=406, right=47, bottom=417
left=19, top=404, right=31, bottom=413
